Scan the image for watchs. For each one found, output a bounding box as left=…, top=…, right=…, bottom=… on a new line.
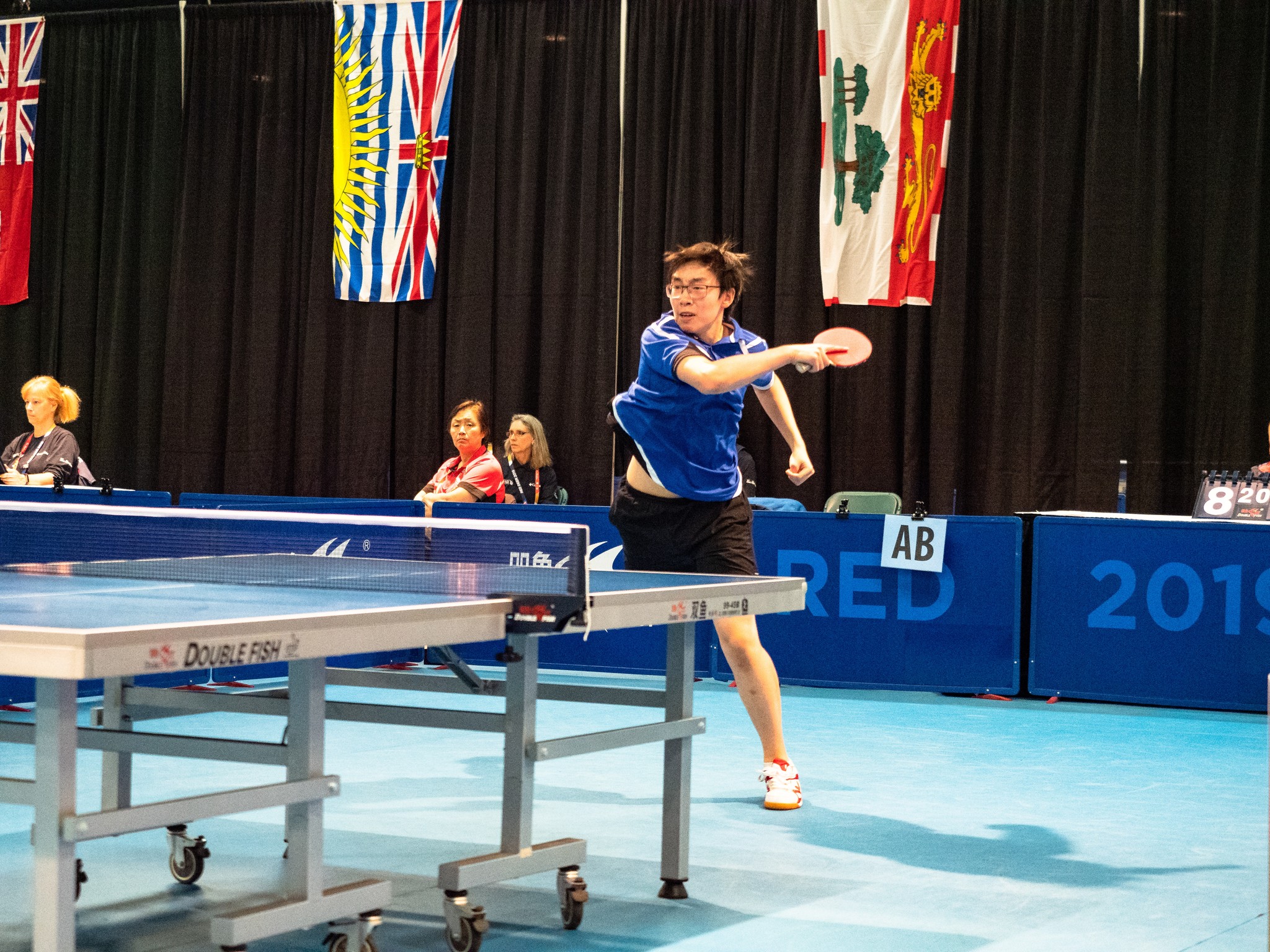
left=22, top=473, right=29, bottom=485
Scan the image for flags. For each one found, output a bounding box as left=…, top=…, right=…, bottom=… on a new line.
left=818, top=0, right=961, bottom=307
left=332, top=0, right=461, bottom=303
left=0, top=15, right=46, bottom=304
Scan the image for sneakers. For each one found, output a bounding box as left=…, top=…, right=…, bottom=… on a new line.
left=757, top=758, right=803, bottom=810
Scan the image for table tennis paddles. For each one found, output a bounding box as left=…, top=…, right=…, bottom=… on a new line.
left=795, top=326, right=873, bottom=374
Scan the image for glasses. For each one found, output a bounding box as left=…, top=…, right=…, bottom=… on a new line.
left=665, top=283, right=725, bottom=298
left=506, top=431, right=531, bottom=436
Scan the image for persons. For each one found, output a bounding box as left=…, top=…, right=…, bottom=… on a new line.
left=1251, top=424, right=1270, bottom=478
left=0, top=374, right=81, bottom=485
left=736, top=443, right=756, bottom=497
left=501, top=415, right=558, bottom=505
left=413, top=398, right=506, bottom=519
left=610, top=242, right=848, bottom=811
